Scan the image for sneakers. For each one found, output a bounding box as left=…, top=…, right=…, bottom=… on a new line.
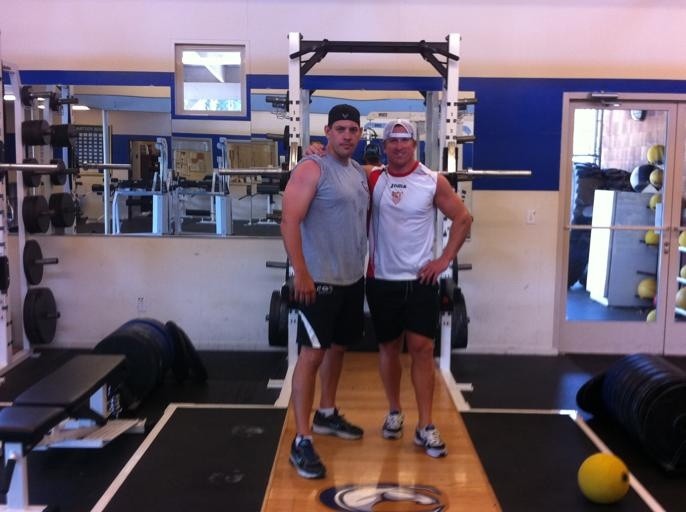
left=311, top=407, right=362, bottom=439
left=415, top=423, right=448, bottom=457
left=289, top=433, right=324, bottom=478
left=382, top=410, right=405, bottom=439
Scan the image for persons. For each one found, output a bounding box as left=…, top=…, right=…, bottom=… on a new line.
left=302, top=118, right=475, bottom=463
left=276, top=102, right=377, bottom=480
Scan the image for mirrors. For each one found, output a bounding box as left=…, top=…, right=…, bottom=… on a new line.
left=174, top=42, right=247, bottom=117
left=563, top=106, right=670, bottom=324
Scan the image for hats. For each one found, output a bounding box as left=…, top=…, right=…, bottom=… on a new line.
left=328, top=104, right=360, bottom=124
left=383, top=121, right=416, bottom=140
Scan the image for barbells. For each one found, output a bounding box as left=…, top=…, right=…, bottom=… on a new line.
left=265, top=291, right=467, bottom=350
left=218, top=141, right=532, bottom=193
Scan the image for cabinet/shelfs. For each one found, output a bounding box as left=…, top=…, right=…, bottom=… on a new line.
left=585, top=189, right=664, bottom=320
